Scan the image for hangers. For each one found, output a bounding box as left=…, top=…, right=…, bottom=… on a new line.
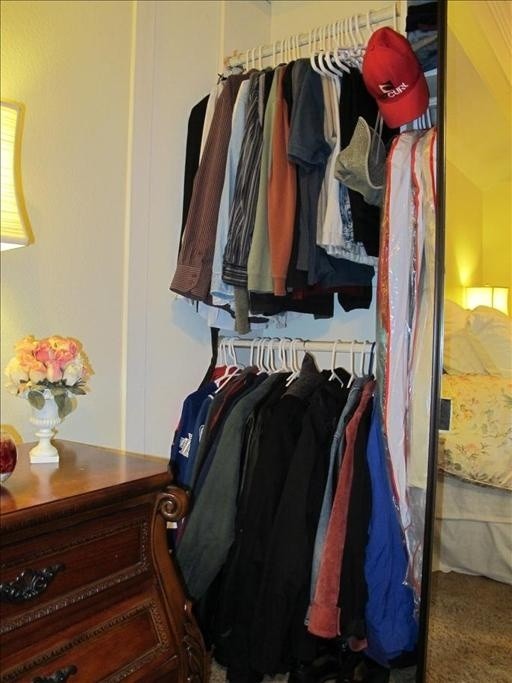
left=196, top=334, right=385, bottom=398
left=221, top=2, right=407, bottom=79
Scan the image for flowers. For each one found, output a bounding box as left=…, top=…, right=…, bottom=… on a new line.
left=3, top=333, right=97, bottom=420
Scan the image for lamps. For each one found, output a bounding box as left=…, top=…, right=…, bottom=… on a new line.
left=1, top=95, right=39, bottom=485
left=463, top=284, right=509, bottom=315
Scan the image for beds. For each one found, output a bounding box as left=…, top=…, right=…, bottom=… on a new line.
left=431, top=298, right=512, bottom=586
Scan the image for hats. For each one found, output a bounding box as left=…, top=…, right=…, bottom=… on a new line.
left=362, top=26, right=430, bottom=129
left=334, top=117, right=389, bottom=208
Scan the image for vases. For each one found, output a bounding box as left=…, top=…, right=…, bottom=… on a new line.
left=29, top=388, right=61, bottom=463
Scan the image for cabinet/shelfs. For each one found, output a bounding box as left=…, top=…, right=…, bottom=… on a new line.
left=0, top=434, right=211, bottom=682
